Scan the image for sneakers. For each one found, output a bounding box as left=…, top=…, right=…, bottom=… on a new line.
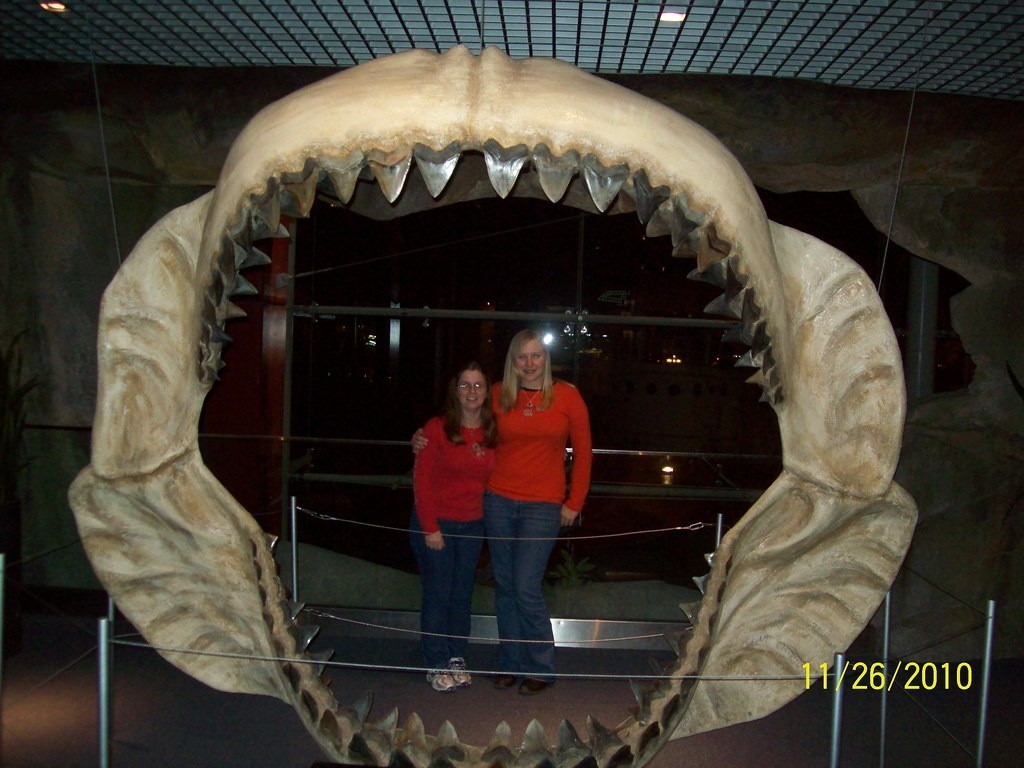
left=427, top=657, right=472, bottom=693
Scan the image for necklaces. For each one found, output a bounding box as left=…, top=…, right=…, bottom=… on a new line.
left=519, top=382, right=544, bottom=408
left=464, top=414, right=483, bottom=438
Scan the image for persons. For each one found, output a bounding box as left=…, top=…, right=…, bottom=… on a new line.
left=412, top=326, right=593, bottom=697
left=413, top=359, right=497, bottom=694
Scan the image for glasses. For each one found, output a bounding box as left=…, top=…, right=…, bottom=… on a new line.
left=457, top=383, right=487, bottom=390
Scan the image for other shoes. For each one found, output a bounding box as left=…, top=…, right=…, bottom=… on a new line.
left=494, top=674, right=523, bottom=689
left=519, top=678, right=555, bottom=694
